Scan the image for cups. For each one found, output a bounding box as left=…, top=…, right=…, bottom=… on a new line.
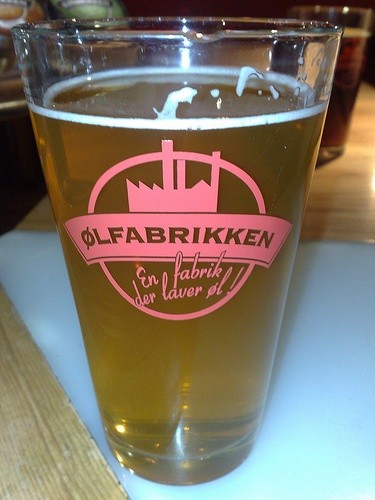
left=9, top=15, right=347, bottom=487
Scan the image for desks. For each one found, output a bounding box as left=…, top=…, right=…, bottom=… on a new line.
left=1, top=86, right=373, bottom=499
